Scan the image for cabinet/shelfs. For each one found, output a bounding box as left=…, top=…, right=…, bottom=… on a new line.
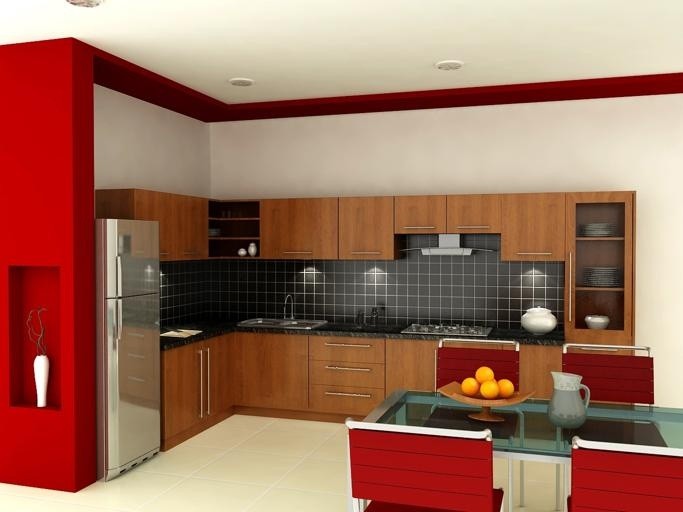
left=393, top=194, right=501, bottom=235
left=233, top=331, right=308, bottom=419
left=499, top=343, right=561, bottom=442
left=259, top=195, right=402, bottom=262
left=500, top=193, right=564, bottom=264
left=385, top=335, right=499, bottom=428
left=308, top=334, right=386, bottom=424
left=160, top=332, right=233, bottom=453
left=208, top=198, right=260, bottom=258
left=95, top=185, right=210, bottom=262
left=565, top=190, right=636, bottom=443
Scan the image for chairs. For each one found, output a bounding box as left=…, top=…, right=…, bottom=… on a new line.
left=420, top=337, right=527, bottom=508
left=563, top=433, right=682, bottom=511
left=558, top=341, right=672, bottom=511
left=338, top=416, right=508, bottom=511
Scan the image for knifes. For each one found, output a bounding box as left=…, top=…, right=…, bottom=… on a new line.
left=164, top=327, right=193, bottom=337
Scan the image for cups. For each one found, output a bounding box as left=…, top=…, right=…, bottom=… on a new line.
left=584, top=314, right=610, bottom=329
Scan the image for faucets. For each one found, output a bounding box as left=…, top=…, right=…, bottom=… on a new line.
left=282, top=294, right=295, bottom=320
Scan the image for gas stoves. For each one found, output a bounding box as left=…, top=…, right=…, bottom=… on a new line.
left=400, top=323, right=492, bottom=337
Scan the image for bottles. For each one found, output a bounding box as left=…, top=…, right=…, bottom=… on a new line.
left=521, top=305, right=558, bottom=336
left=370, top=308, right=379, bottom=327
left=238, top=248, right=248, bottom=256
left=248, top=243, right=257, bottom=258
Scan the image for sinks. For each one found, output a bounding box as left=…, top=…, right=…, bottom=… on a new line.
left=279, top=319, right=329, bottom=330
left=236, top=317, right=285, bottom=328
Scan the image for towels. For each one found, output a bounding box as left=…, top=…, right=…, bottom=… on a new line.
left=160, top=326, right=202, bottom=340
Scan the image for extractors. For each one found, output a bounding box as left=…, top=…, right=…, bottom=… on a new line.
left=398, top=234, right=501, bottom=256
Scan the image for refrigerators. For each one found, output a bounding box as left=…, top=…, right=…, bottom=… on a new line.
left=96, top=218, right=161, bottom=482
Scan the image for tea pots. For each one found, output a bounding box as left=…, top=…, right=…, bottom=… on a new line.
left=547, top=371, right=591, bottom=429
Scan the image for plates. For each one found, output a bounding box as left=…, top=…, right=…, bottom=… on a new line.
left=582, top=265, right=622, bottom=288
left=208, top=229, right=221, bottom=236
left=160, top=329, right=203, bottom=339
left=578, top=221, right=618, bottom=237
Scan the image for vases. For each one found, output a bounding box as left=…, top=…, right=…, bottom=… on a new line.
left=544, top=367, right=592, bottom=428
left=520, top=306, right=559, bottom=338
left=31, top=351, right=52, bottom=409
left=581, top=310, right=612, bottom=330
